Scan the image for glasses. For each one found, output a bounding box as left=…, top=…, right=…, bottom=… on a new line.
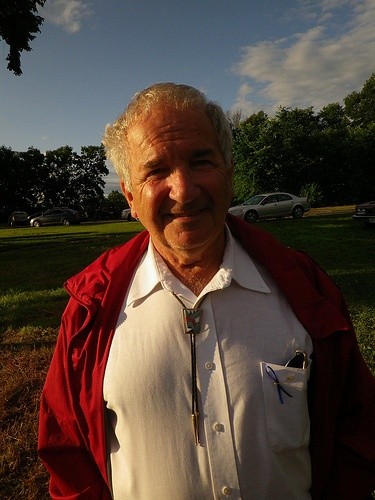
left=284, top=350, right=309, bottom=370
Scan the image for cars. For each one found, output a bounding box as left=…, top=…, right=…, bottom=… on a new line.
left=121, top=209, right=136, bottom=222
left=351, top=201, right=375, bottom=228
left=228, top=192, right=311, bottom=224
left=8, top=211, right=31, bottom=226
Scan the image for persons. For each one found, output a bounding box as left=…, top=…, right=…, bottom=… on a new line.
left=38, top=83, right=375, bottom=500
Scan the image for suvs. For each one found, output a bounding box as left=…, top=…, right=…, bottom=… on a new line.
left=29, top=206, right=80, bottom=228
